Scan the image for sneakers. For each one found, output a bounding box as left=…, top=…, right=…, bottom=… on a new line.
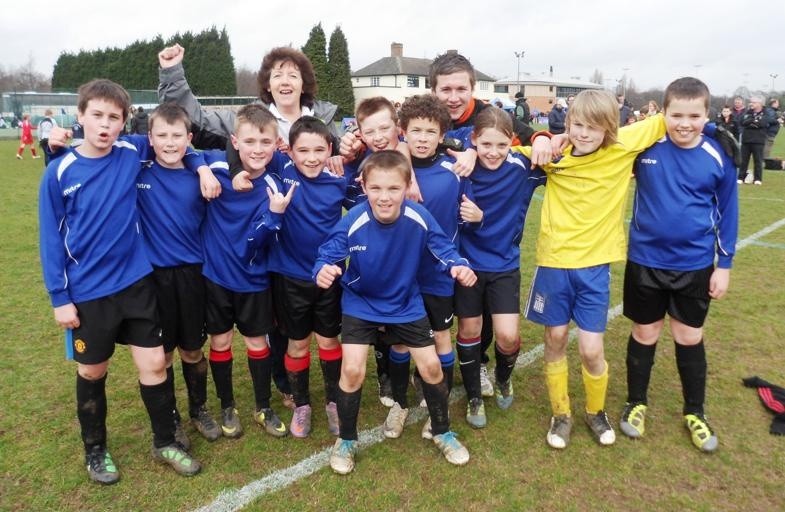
left=32, top=155, right=40, bottom=159
left=409, top=375, right=423, bottom=390
left=173, top=418, right=193, bottom=452
left=330, top=437, right=359, bottom=475
left=421, top=410, right=450, bottom=440
left=619, top=401, right=648, bottom=438
left=16, top=153, right=23, bottom=159
left=191, top=404, right=222, bottom=441
left=753, top=180, right=762, bottom=186
left=152, top=439, right=201, bottom=477
left=279, top=394, right=295, bottom=409
left=495, top=377, right=514, bottom=410
left=545, top=413, right=575, bottom=449
left=377, top=375, right=395, bottom=407
left=584, top=409, right=616, bottom=444
left=254, top=406, right=291, bottom=438
left=433, top=432, right=470, bottom=465
left=736, top=178, right=745, bottom=186
left=289, top=404, right=314, bottom=438
left=682, top=412, right=719, bottom=452
left=479, top=362, right=495, bottom=398
left=466, top=397, right=487, bottom=429
left=85, top=447, right=121, bottom=487
left=325, top=402, right=340, bottom=436
left=220, top=404, right=244, bottom=439
left=382, top=400, right=409, bottom=438
left=420, top=398, right=427, bottom=407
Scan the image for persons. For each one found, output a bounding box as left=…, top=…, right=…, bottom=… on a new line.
left=338, top=51, right=553, bottom=398
left=624, top=113, right=638, bottom=125
left=615, top=98, right=632, bottom=127
left=158, top=43, right=345, bottom=409
left=200, top=105, right=296, bottom=438
left=37, top=78, right=222, bottom=485
left=731, top=95, right=748, bottom=127
left=38, top=104, right=223, bottom=452
left=551, top=77, right=739, bottom=453
left=393, top=107, right=564, bottom=429
left=355, top=97, right=478, bottom=407
left=130, top=106, right=148, bottom=135
left=381, top=92, right=484, bottom=441
left=16, top=112, right=41, bottom=160
left=495, top=102, right=503, bottom=107
left=717, top=104, right=738, bottom=141
left=226, top=115, right=351, bottom=439
left=71, top=114, right=84, bottom=139
left=648, top=100, right=659, bottom=117
left=737, top=96, right=768, bottom=186
left=443, top=88, right=743, bottom=449
left=513, top=90, right=530, bottom=125
left=310, top=151, right=478, bottom=476
left=548, top=98, right=567, bottom=135
left=566, top=94, right=574, bottom=105
left=638, top=107, right=649, bottom=120
left=618, top=94, right=629, bottom=106
left=36, top=109, right=60, bottom=167
left=763, top=99, right=780, bottom=158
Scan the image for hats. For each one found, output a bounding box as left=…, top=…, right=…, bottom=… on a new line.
left=557, top=98, right=567, bottom=108
left=515, top=92, right=523, bottom=97
